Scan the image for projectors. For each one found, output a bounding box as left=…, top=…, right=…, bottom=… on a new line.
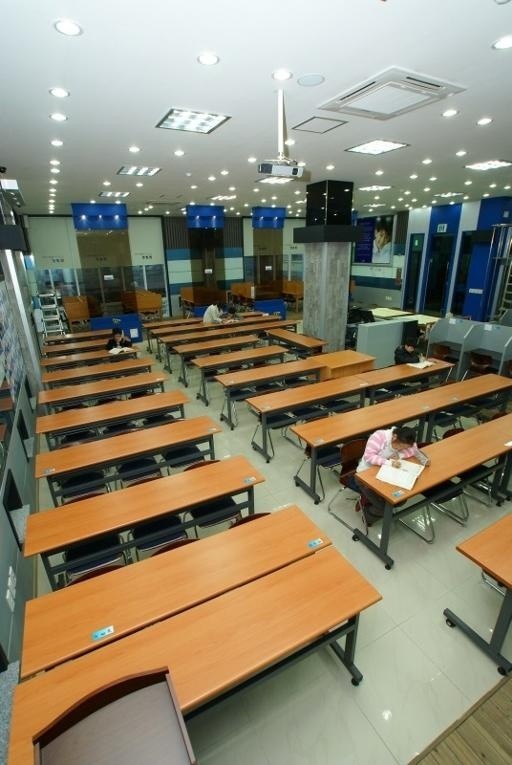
left=257, top=157, right=304, bottom=178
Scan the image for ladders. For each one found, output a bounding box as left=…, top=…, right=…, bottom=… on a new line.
left=36, top=293, right=65, bottom=358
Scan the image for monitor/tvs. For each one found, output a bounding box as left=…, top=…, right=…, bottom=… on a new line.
left=351, top=212, right=398, bottom=266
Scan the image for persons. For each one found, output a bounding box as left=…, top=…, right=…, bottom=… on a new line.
left=203, top=299, right=224, bottom=324
left=105, top=328, right=131, bottom=350
left=355, top=426, right=418, bottom=526
left=372, top=223, right=391, bottom=264
left=220, top=307, right=240, bottom=320
left=394, top=337, right=429, bottom=387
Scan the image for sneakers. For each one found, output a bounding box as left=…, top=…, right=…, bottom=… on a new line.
left=356, top=493, right=368, bottom=512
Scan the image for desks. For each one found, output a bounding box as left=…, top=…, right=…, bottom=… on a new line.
left=8, top=278, right=512, bottom=765
